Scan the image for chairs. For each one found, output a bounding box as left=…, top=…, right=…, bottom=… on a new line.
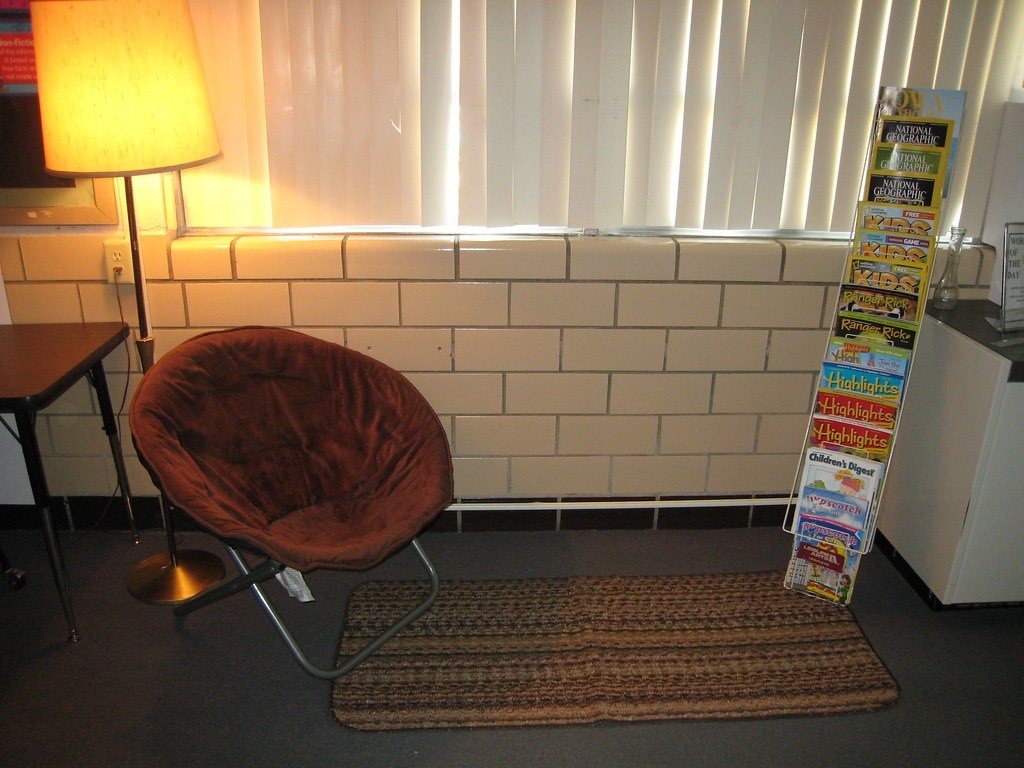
left=127, top=326, right=455, bottom=680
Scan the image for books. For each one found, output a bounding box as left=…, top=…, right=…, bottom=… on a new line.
left=877, top=86, right=968, bottom=199
left=790, top=116, right=955, bottom=554
left=784, top=483, right=869, bottom=609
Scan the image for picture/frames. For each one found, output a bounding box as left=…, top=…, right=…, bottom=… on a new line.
left=0, top=1, right=120, bottom=226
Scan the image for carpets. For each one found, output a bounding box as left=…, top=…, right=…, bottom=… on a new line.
left=328, top=568, right=900, bottom=732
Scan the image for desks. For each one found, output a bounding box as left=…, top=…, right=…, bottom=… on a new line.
left=0, top=320, right=143, bottom=644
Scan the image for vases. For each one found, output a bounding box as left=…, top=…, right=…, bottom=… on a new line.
left=933, top=227, right=967, bottom=311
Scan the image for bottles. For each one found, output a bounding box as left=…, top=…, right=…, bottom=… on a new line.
left=932, top=227, right=966, bottom=310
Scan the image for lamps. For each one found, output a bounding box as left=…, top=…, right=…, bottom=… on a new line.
left=28, top=0, right=226, bottom=608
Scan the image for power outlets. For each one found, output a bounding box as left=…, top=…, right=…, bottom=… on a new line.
left=104, top=239, right=136, bottom=284
